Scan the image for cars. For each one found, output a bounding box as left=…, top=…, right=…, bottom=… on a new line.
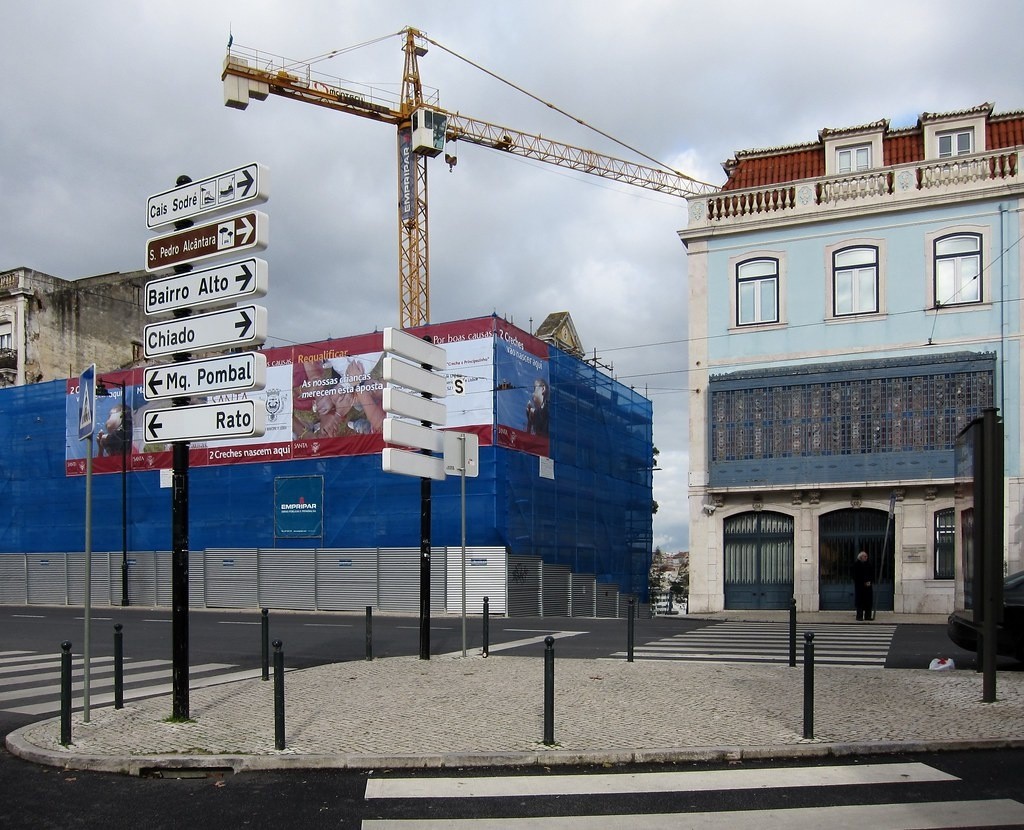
left=999, top=570, right=1023, bottom=662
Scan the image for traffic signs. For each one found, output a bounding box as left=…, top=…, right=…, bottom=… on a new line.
left=143, top=399, right=266, bottom=443
left=145, top=162, right=270, bottom=232
left=145, top=210, right=269, bottom=274
left=144, top=257, right=269, bottom=316
left=143, top=350, right=266, bottom=401
left=143, top=305, right=267, bottom=359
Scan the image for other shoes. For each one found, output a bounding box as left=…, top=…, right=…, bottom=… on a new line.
left=864, top=616, right=873, bottom=620
left=856, top=616, right=863, bottom=621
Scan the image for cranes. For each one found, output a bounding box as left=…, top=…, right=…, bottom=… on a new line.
left=223, top=24, right=722, bottom=333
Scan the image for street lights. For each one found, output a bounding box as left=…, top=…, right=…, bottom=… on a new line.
left=96, top=376, right=130, bottom=608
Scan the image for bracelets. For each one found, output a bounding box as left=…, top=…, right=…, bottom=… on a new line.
left=298, top=429, right=308, bottom=439
left=335, top=412, right=341, bottom=417
left=353, top=382, right=364, bottom=391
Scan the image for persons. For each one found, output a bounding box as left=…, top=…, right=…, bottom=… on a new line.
left=292, top=352, right=385, bottom=438
left=852, top=552, right=874, bottom=621
left=96, top=405, right=124, bottom=456
left=525, top=378, right=549, bottom=436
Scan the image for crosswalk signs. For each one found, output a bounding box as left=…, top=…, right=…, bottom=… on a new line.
left=78, top=364, right=95, bottom=442
